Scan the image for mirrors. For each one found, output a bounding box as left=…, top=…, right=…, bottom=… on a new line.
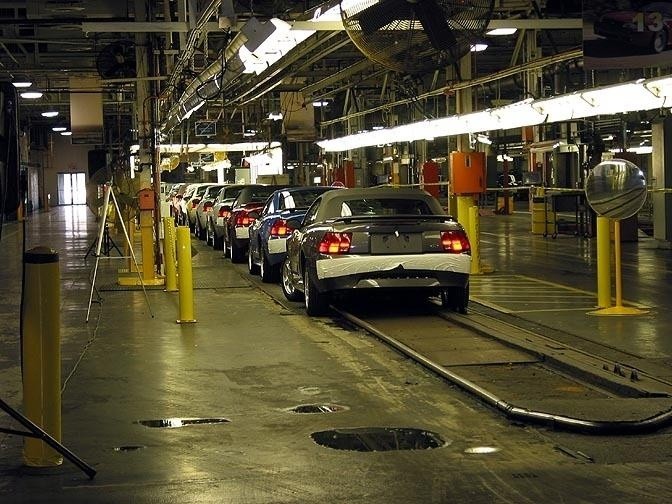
left=585, top=158, right=647, bottom=220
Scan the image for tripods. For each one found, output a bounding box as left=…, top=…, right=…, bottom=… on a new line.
left=84, top=184, right=125, bottom=260
left=85, top=183, right=154, bottom=323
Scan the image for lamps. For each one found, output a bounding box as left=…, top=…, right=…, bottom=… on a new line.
left=130, top=0, right=672, bottom=184
left=11, top=77, right=72, bottom=135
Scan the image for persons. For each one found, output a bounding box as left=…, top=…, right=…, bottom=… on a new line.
left=169, top=191, right=189, bottom=228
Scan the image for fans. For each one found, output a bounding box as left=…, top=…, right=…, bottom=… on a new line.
left=97, top=40, right=143, bottom=84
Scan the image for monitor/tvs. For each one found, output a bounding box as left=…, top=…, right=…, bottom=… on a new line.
left=195, top=121, right=217, bottom=137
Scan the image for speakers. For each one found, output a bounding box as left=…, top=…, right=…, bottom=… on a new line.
left=88, top=150, right=113, bottom=183
